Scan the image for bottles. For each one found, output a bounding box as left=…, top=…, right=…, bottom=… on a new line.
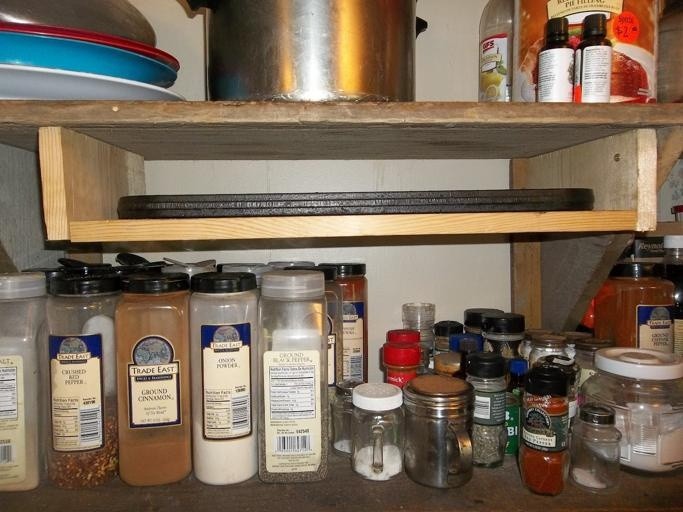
left=351, top=232, right=682, bottom=498
left=0, top=257, right=371, bottom=499
left=476, top=0, right=512, bottom=103
left=537, top=16, right=574, bottom=105
left=575, top=13, right=610, bottom=106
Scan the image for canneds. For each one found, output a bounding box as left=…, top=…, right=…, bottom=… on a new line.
left=592, top=262, right=676, bottom=352
left=518, top=331, right=615, bottom=370
left=579, top=347, right=683, bottom=473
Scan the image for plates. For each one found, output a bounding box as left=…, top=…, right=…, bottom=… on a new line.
left=0, top=21, right=183, bottom=107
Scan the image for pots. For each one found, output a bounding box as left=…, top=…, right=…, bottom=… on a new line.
left=184, top=0, right=429, bottom=107
